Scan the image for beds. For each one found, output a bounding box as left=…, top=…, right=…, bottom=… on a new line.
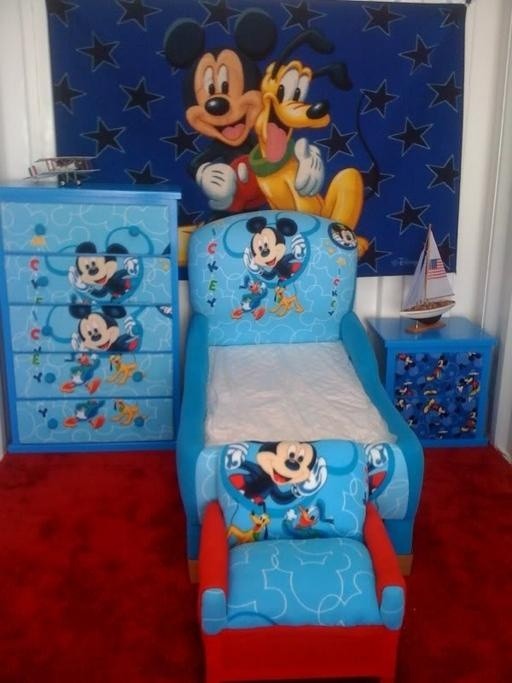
left=177, top=208, right=425, bottom=583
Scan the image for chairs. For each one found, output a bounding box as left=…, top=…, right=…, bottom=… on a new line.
left=195, top=437, right=407, bottom=682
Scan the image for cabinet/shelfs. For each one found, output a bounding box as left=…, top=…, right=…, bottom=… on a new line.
left=0, top=179, right=182, bottom=455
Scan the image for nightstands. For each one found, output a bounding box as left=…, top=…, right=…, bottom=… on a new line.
left=364, top=315, right=498, bottom=449
